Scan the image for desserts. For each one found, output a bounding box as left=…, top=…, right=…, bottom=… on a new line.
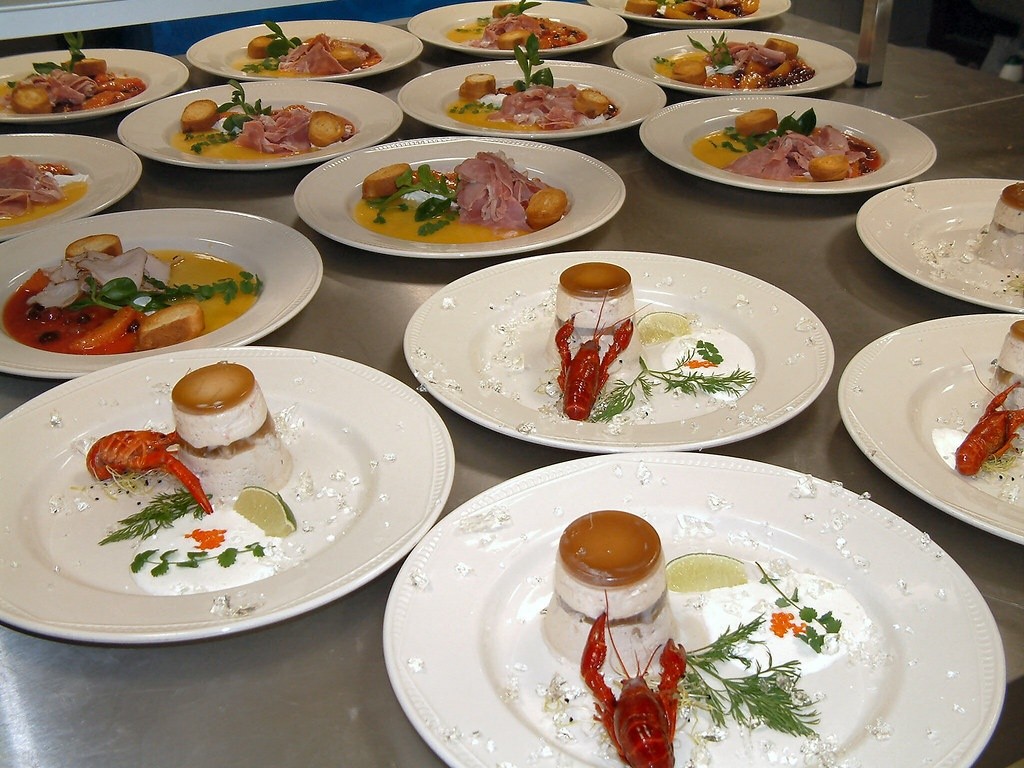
left=991, top=320, right=1024, bottom=412
left=555, top=262, right=635, bottom=334
left=983, top=181, right=1024, bottom=267
left=553, top=510, right=664, bottom=622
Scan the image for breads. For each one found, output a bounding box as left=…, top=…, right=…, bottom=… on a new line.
left=735, top=108, right=778, bottom=136
left=180, top=99, right=220, bottom=132
left=763, top=39, right=799, bottom=59
left=362, top=162, right=410, bottom=199
left=573, top=88, right=610, bottom=117
left=62, top=58, right=107, bottom=76
left=138, top=304, right=205, bottom=349
left=327, top=47, right=361, bottom=70
left=493, top=4, right=518, bottom=18
left=65, top=234, right=123, bottom=259
left=460, top=73, right=497, bottom=99
left=497, top=31, right=531, bottom=49
left=625, top=0, right=658, bottom=15
left=525, top=188, right=567, bottom=228
left=248, top=36, right=275, bottom=58
left=308, top=110, right=343, bottom=146
left=11, top=84, right=52, bottom=114
left=671, top=60, right=706, bottom=84
left=805, top=154, right=849, bottom=182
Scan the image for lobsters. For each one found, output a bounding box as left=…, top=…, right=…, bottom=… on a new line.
left=581, top=590, right=687, bottom=768
left=555, top=291, right=652, bottom=420
left=956, top=348, right=1024, bottom=475
left=86, top=430, right=213, bottom=515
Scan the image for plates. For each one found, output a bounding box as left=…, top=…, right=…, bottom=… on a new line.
left=118, top=81, right=402, bottom=169
left=407, top=0, right=628, bottom=59
left=0, top=208, right=323, bottom=379
left=837, top=314, right=1024, bottom=545
left=0, top=133, right=142, bottom=242
left=293, top=136, right=626, bottom=259
left=587, top=0, right=791, bottom=28
left=0, top=49, right=189, bottom=125
left=612, top=28, right=857, bottom=95
left=186, top=20, right=423, bottom=81
left=397, top=58, right=667, bottom=142
left=0, top=346, right=456, bottom=644
left=382, top=451, right=1008, bottom=768
left=403, top=251, right=834, bottom=453
left=855, top=177, right=1024, bottom=314
left=639, top=95, right=937, bottom=194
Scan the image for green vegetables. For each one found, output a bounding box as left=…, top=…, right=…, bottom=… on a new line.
left=7, top=0, right=842, bottom=741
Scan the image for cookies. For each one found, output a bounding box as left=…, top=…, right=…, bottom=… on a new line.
left=171, top=362, right=278, bottom=460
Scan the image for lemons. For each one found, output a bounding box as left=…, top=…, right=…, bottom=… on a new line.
left=233, top=486, right=297, bottom=538
left=663, top=552, right=747, bottom=592
left=636, top=312, right=692, bottom=345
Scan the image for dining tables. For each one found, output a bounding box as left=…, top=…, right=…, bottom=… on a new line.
left=0, top=21, right=1024, bottom=768
